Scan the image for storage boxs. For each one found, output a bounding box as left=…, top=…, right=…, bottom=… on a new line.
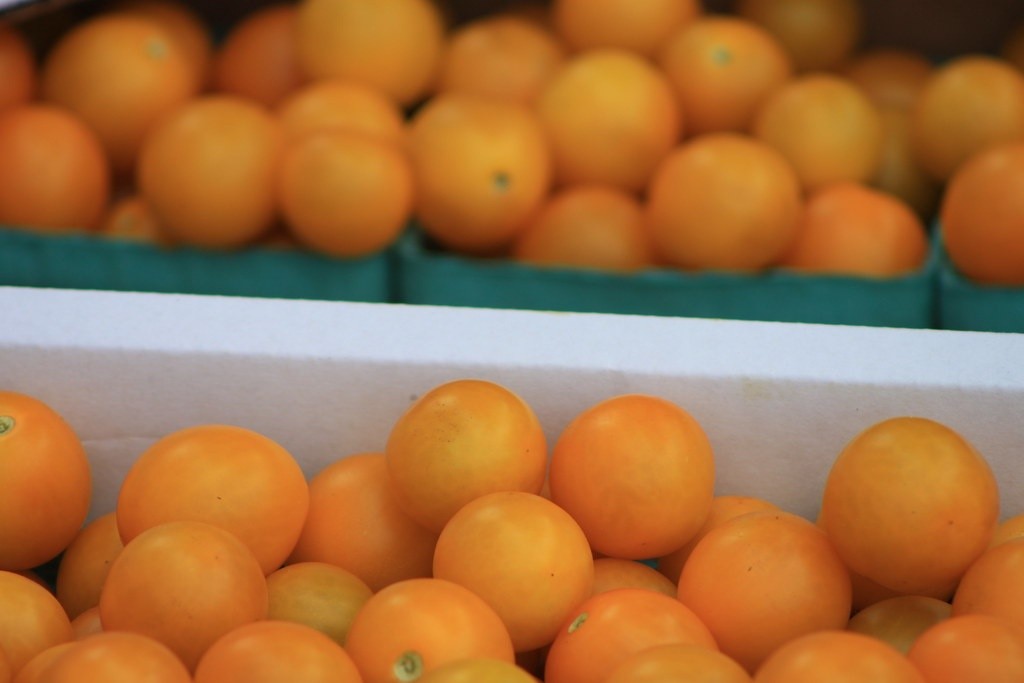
left=4, top=279, right=1024, bottom=534
left=2, top=0, right=1020, bottom=335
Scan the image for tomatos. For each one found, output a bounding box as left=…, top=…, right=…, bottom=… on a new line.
left=2, top=0, right=1023, bottom=288
left=2, top=378, right=1021, bottom=683
